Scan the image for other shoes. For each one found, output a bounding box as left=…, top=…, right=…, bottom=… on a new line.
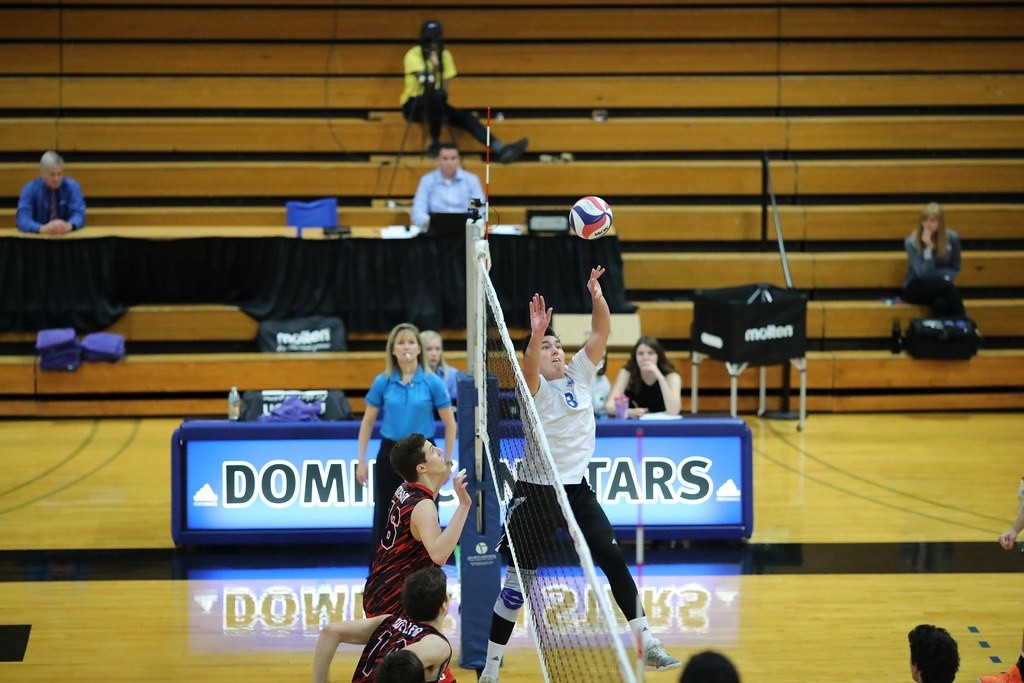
left=498, top=138, right=530, bottom=164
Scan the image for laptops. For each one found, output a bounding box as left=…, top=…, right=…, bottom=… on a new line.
left=421, top=213, right=469, bottom=236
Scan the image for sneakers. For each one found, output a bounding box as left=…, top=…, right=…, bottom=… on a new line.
left=644, top=639, right=682, bottom=671
left=479, top=674, right=500, bottom=683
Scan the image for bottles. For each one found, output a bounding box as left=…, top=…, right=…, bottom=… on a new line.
left=599, top=397, right=607, bottom=420
left=892, top=320, right=902, bottom=353
left=229, top=387, right=240, bottom=420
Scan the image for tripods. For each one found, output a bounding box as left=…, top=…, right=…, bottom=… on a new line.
left=383, top=71, right=466, bottom=206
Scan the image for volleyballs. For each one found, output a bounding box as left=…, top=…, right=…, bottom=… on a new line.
left=567, top=195, right=615, bottom=239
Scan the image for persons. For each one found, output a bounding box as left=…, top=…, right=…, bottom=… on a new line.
left=363, top=433, right=473, bottom=683
left=478, top=265, right=681, bottom=683
left=678, top=649, right=741, bottom=683
left=605, top=335, right=682, bottom=418
left=899, top=202, right=966, bottom=318
left=312, top=568, right=457, bottom=683
left=375, top=649, right=426, bottom=683
left=357, top=322, right=457, bottom=580
left=420, top=330, right=459, bottom=421
left=573, top=339, right=611, bottom=415
left=16, top=151, right=86, bottom=235
left=976, top=474, right=1024, bottom=683
left=908, top=624, right=960, bottom=683
left=412, top=143, right=486, bottom=232
left=399, top=20, right=529, bottom=164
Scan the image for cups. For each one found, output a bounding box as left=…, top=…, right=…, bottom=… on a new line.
left=615, top=398, right=629, bottom=420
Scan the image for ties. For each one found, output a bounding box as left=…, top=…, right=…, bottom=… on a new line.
left=49, top=189, right=57, bottom=220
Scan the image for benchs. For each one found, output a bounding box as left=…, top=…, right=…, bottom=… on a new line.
left=0, top=0, right=1024, bottom=418
